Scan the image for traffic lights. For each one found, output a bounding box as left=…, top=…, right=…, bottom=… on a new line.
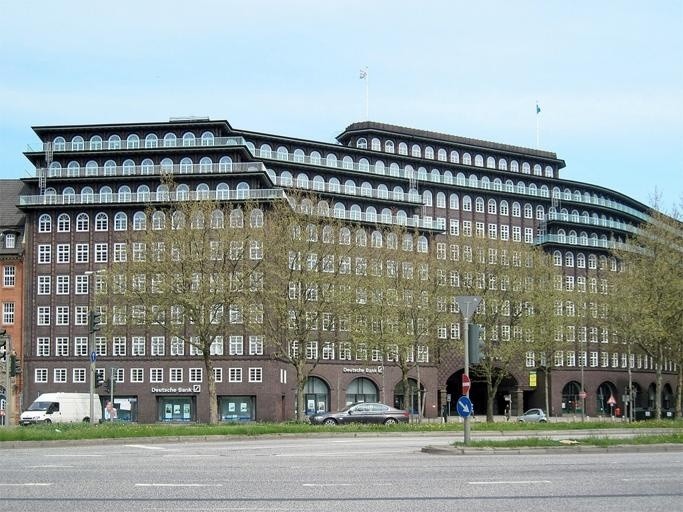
left=10, top=356, right=21, bottom=377
left=88, top=311, right=101, bottom=334
left=0, top=329, right=7, bottom=361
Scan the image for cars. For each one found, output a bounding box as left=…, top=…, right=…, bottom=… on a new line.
left=515, top=408, right=547, bottom=424
left=309, top=402, right=409, bottom=426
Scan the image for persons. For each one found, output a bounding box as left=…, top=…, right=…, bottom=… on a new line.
left=442, top=404, right=448, bottom=423
left=471, top=401, right=475, bottom=418
left=505, top=404, right=511, bottom=422
left=104, top=400, right=117, bottom=419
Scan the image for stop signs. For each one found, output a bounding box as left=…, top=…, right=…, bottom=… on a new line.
left=578, top=392, right=585, bottom=399
left=461, top=375, right=471, bottom=396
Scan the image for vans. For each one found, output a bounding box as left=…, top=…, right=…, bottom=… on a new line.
left=19, top=393, right=102, bottom=425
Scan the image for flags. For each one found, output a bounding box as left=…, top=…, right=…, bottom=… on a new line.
left=537, top=102, right=541, bottom=113
left=359, top=70, right=368, bottom=79
